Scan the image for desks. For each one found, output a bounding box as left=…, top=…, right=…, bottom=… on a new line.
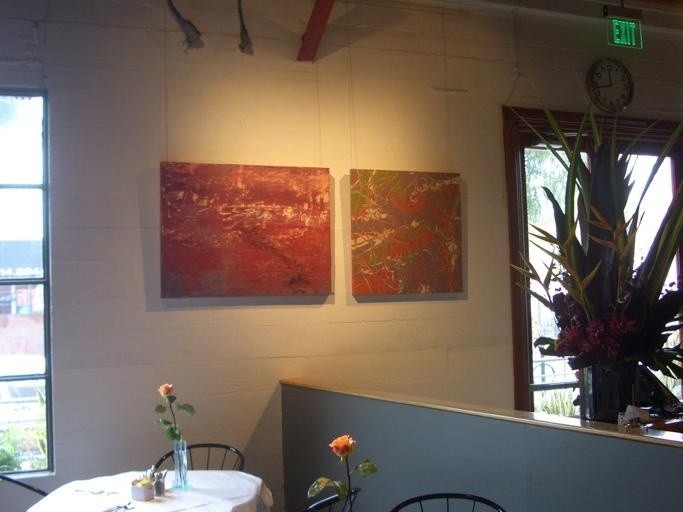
left=26, top=465, right=273, bottom=511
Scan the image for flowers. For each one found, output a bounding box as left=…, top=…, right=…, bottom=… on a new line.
left=308, top=434, right=376, bottom=511
left=151, top=383, right=194, bottom=441
left=508, top=99, right=683, bottom=380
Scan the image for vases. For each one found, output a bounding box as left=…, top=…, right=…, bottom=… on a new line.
left=172, top=438, right=186, bottom=487
left=578, top=365, right=640, bottom=422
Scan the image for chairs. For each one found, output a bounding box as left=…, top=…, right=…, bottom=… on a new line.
left=0, top=472, right=47, bottom=511
left=389, top=491, right=505, bottom=511
left=305, top=486, right=361, bottom=511
left=154, top=443, right=244, bottom=470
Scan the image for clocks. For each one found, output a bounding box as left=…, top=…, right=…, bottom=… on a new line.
left=585, top=55, right=634, bottom=112
